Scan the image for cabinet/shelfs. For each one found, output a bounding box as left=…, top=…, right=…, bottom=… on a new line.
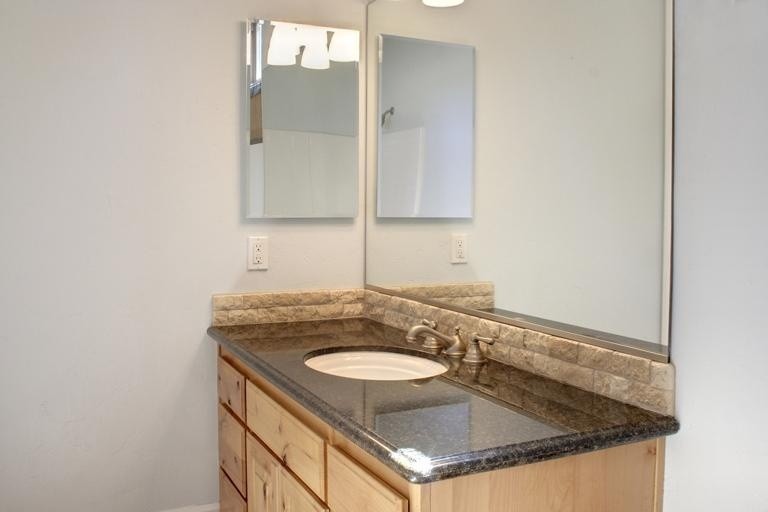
left=217, top=346, right=665, bottom=511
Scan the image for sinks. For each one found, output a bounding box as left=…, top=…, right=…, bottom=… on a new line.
left=304, top=345, right=450, bottom=382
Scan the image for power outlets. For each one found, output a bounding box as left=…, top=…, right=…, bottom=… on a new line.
left=451, top=234, right=468, bottom=263
left=247, top=237, right=270, bottom=270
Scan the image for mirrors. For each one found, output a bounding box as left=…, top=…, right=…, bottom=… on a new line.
left=365, top=0, right=674, bottom=364
left=242, top=19, right=358, bottom=220
left=377, top=34, right=475, bottom=220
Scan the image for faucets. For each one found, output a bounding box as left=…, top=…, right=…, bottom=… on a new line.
left=405, top=325, right=466, bottom=361
